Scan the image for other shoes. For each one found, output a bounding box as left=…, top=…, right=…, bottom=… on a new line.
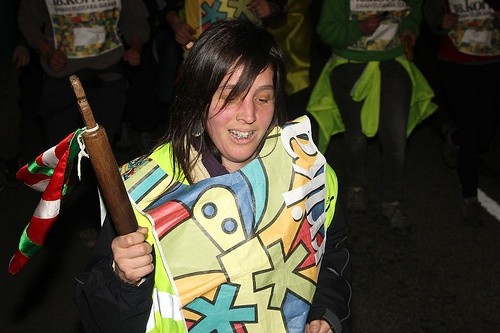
left=76, top=229, right=99, bottom=250
left=381, top=202, right=411, bottom=230
left=461, top=199, right=489, bottom=227
left=344, top=192, right=370, bottom=237
left=2, top=160, right=21, bottom=187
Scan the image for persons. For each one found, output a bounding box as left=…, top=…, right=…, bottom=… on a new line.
left=11, top=0, right=500, bottom=248
left=77, top=18, right=353, bottom=333
